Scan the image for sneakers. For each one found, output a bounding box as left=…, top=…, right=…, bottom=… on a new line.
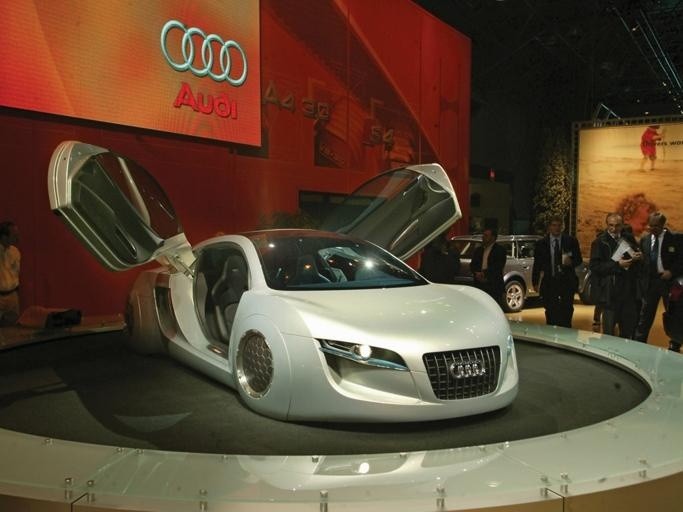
left=591, top=318, right=600, bottom=332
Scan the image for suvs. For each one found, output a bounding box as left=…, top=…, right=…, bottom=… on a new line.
left=442, top=223, right=596, bottom=305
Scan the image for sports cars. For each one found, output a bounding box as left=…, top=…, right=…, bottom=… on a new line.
left=44, top=130, right=524, bottom=422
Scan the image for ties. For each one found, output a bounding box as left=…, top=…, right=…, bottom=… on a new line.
left=648, top=236, right=658, bottom=275
left=553, top=238, right=562, bottom=281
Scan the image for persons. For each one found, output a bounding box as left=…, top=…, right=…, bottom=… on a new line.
left=589, top=212, right=682, bottom=353
left=639, top=125, right=662, bottom=172
left=532, top=215, right=583, bottom=328
left=469, top=229, right=506, bottom=310
left=1, top=221, right=21, bottom=324
left=416, top=228, right=461, bottom=284
